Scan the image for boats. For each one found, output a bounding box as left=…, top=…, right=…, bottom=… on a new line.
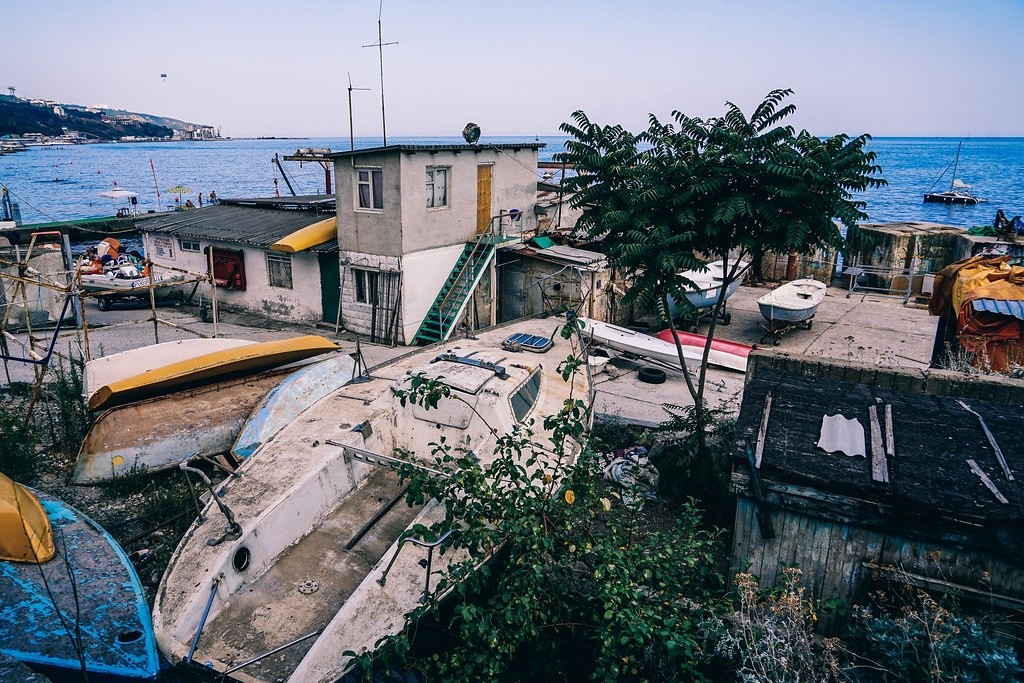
left=656, top=259, right=754, bottom=322
left=653, top=328, right=765, bottom=372
left=577, top=315, right=704, bottom=371
left=0, top=471, right=161, bottom=683
left=920, top=141, right=985, bottom=206
left=148, top=305, right=594, bottom=683
left=756, top=277, right=827, bottom=324
left=227, top=353, right=366, bottom=467
left=68, top=335, right=338, bottom=485
left=72, top=256, right=184, bottom=299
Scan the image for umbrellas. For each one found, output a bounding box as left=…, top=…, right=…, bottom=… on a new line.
left=163, top=185, right=192, bottom=202
left=100, top=188, right=139, bottom=200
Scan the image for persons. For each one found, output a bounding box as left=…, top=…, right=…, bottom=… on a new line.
left=128, top=197, right=137, bottom=218
left=186, top=200, right=195, bottom=208
left=197, top=192, right=202, bottom=208
left=210, top=190, right=216, bottom=204
